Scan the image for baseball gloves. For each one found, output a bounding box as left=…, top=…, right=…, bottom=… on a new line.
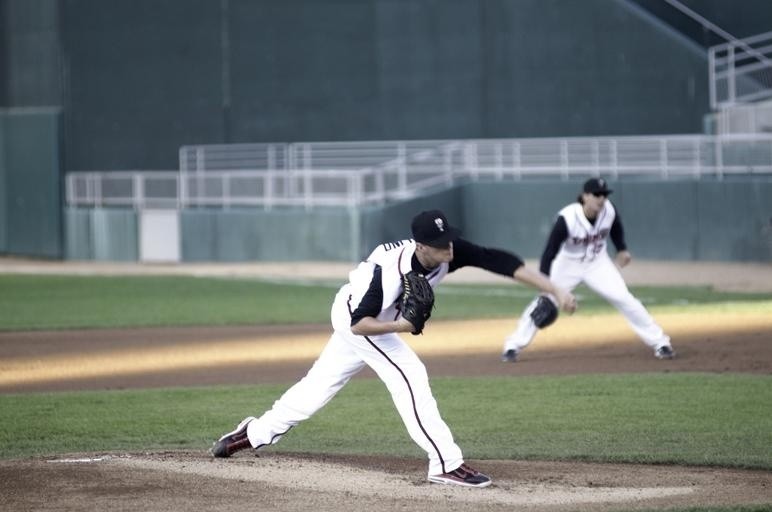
left=395, top=271, right=434, bottom=334
left=529, top=296, right=558, bottom=329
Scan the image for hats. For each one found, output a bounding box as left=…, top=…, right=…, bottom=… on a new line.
left=585, top=179, right=613, bottom=193
left=412, top=210, right=462, bottom=247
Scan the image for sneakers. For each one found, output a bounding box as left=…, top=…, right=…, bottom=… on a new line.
left=427, top=462, right=491, bottom=487
left=211, top=417, right=256, bottom=457
left=655, top=346, right=675, bottom=359
left=502, top=349, right=519, bottom=362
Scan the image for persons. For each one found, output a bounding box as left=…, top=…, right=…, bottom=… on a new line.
left=208, top=209, right=581, bottom=491
left=501, top=176, right=679, bottom=362
